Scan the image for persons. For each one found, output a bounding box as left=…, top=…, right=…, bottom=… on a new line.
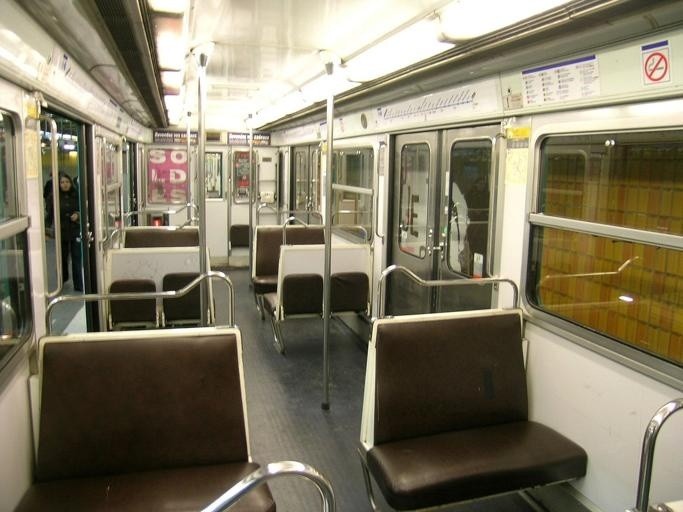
left=465, top=178, right=485, bottom=277
left=46, top=172, right=84, bottom=291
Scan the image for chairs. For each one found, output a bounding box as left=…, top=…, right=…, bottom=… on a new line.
left=229, top=222, right=252, bottom=251
left=100, top=225, right=208, bottom=330
left=254, top=221, right=370, bottom=357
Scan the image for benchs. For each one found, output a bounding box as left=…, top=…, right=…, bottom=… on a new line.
left=356, top=259, right=597, bottom=512
left=11, top=268, right=287, bottom=511
